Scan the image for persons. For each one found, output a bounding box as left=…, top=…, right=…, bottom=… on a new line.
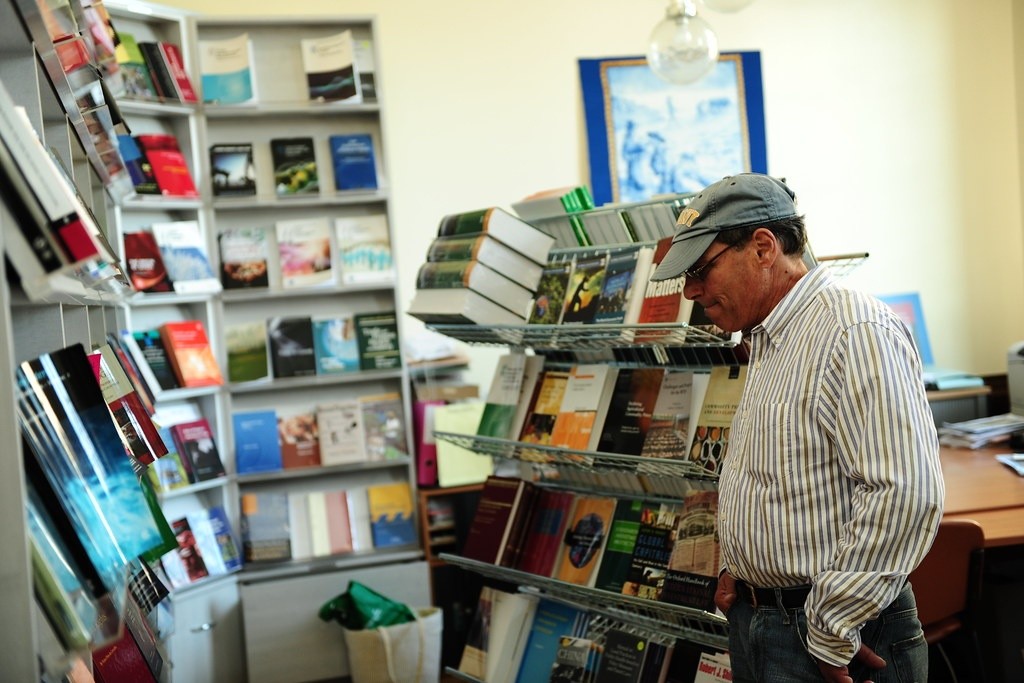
left=649, top=174, right=946, bottom=683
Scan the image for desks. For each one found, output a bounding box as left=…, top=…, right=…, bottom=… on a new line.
left=940, top=424, right=1024, bottom=550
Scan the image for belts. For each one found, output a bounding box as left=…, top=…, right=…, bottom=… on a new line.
left=732, top=581, right=809, bottom=609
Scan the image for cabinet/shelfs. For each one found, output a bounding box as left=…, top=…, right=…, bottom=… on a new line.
left=179, top=13, right=438, bottom=683
left=0, top=0, right=252, bottom=683
left=404, top=178, right=821, bottom=683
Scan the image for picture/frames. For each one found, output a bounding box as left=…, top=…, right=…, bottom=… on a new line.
left=578, top=51, right=767, bottom=207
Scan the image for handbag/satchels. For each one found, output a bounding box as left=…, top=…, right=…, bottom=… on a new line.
left=317, top=579, right=415, bottom=631
left=341, top=606, right=444, bottom=683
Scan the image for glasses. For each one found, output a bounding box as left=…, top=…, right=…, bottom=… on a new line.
left=680, top=235, right=749, bottom=282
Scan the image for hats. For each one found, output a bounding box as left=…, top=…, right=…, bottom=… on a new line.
left=649, top=173, right=797, bottom=282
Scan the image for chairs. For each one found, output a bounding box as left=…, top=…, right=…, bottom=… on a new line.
left=889, top=519, right=987, bottom=683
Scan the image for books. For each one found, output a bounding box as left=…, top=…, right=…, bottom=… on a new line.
left=923, top=371, right=1024, bottom=477
left=405, top=183, right=749, bottom=683
left=0, top=0, right=243, bottom=683
left=198, top=28, right=420, bottom=563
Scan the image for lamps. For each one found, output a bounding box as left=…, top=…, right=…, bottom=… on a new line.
left=645, top=0, right=718, bottom=85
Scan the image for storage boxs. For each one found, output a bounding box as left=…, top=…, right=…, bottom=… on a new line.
left=341, top=606, right=444, bottom=683
